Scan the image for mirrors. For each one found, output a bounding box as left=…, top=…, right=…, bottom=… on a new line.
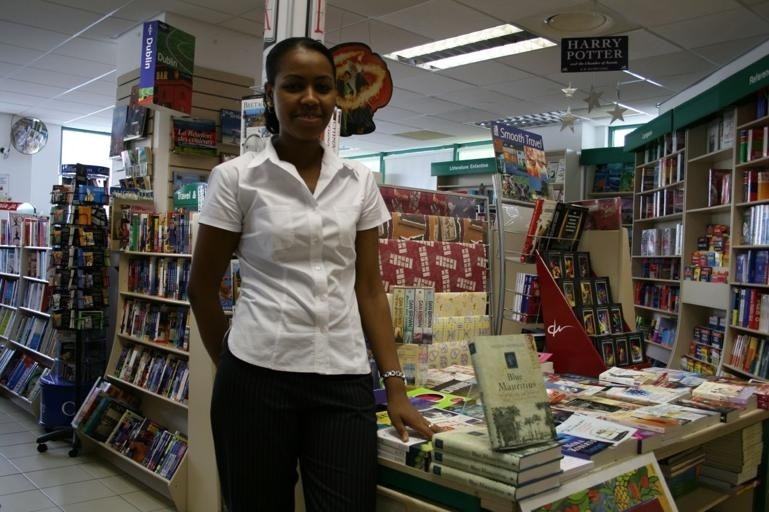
left=10, top=116, right=49, bottom=155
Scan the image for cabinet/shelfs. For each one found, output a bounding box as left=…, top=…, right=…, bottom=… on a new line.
left=630, top=122, right=688, bottom=367
left=75, top=237, right=239, bottom=512
left=0, top=218, right=22, bottom=342
left=536, top=248, right=652, bottom=377
left=665, top=107, right=736, bottom=378
left=715, top=98, right=768, bottom=381
left=1, top=221, right=56, bottom=419
left=377, top=409, right=769, bottom=511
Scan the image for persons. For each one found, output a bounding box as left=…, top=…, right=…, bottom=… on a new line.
left=185, top=37, right=453, bottom=512
